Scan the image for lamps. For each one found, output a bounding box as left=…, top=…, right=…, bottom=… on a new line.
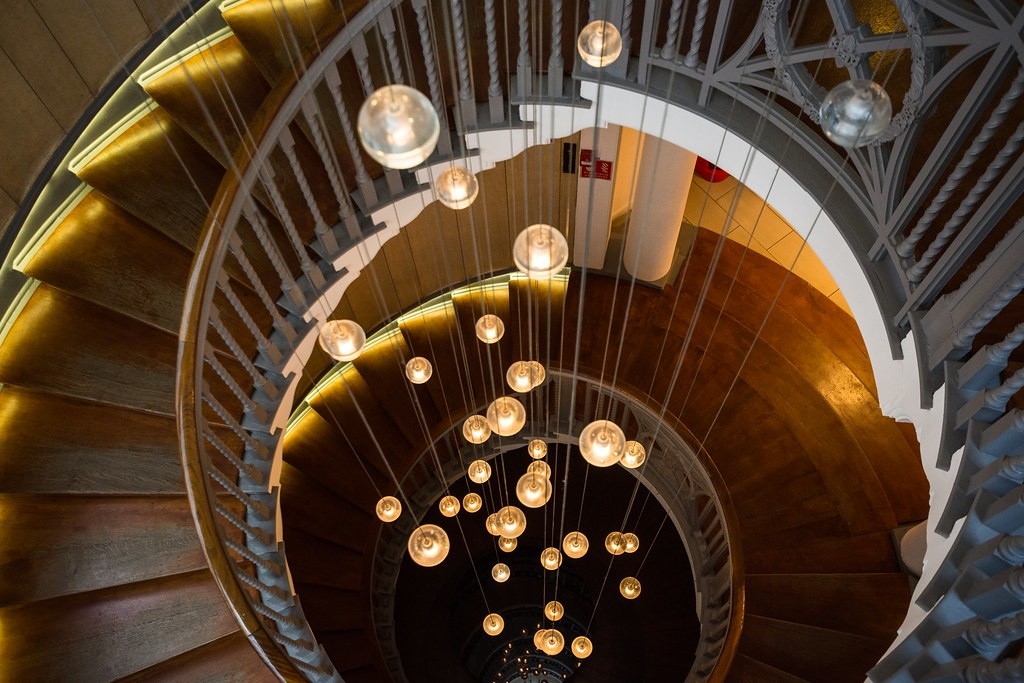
left=83, top=0, right=926, bottom=659
